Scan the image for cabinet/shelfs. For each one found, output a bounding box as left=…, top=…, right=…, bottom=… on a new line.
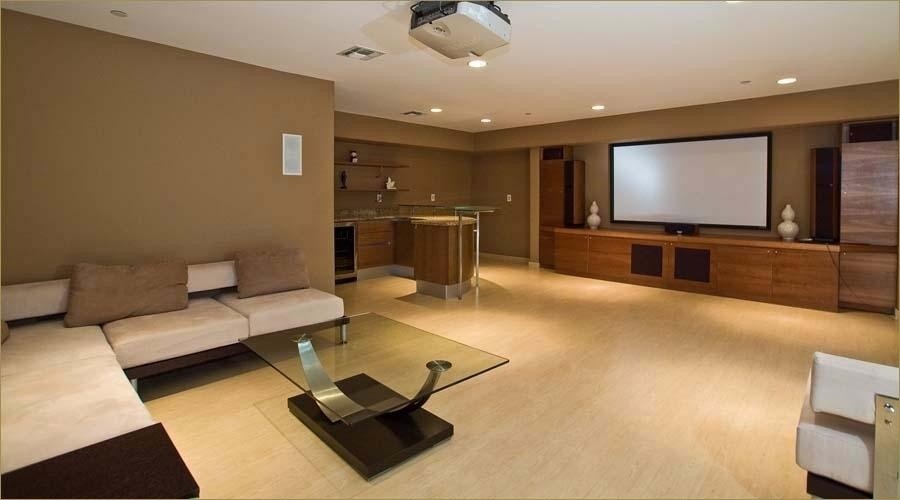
left=839, top=138, right=899, bottom=316
left=553, top=231, right=627, bottom=284
left=538, top=157, right=585, bottom=271
left=334, top=160, right=411, bottom=193
left=626, top=237, right=717, bottom=297
left=715, top=245, right=839, bottom=313
left=358, top=222, right=393, bottom=269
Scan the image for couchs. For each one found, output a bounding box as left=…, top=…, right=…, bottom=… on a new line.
left=0, top=258, right=349, bottom=499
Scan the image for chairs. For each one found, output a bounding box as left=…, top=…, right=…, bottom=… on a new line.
left=794, top=350, right=899, bottom=500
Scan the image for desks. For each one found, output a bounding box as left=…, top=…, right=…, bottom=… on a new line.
left=393, top=213, right=478, bottom=303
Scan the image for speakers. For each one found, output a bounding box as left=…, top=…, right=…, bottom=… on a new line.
left=809, top=146, right=840, bottom=242
left=564, top=160, right=585, bottom=227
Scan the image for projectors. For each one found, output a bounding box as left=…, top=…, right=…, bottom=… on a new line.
left=408, top=1, right=513, bottom=60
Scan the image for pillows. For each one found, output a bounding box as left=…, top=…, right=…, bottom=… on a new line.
left=59, top=261, right=189, bottom=328
left=231, top=243, right=311, bottom=299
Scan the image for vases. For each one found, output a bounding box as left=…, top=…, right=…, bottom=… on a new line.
left=778, top=204, right=800, bottom=240
left=587, top=200, right=601, bottom=230
